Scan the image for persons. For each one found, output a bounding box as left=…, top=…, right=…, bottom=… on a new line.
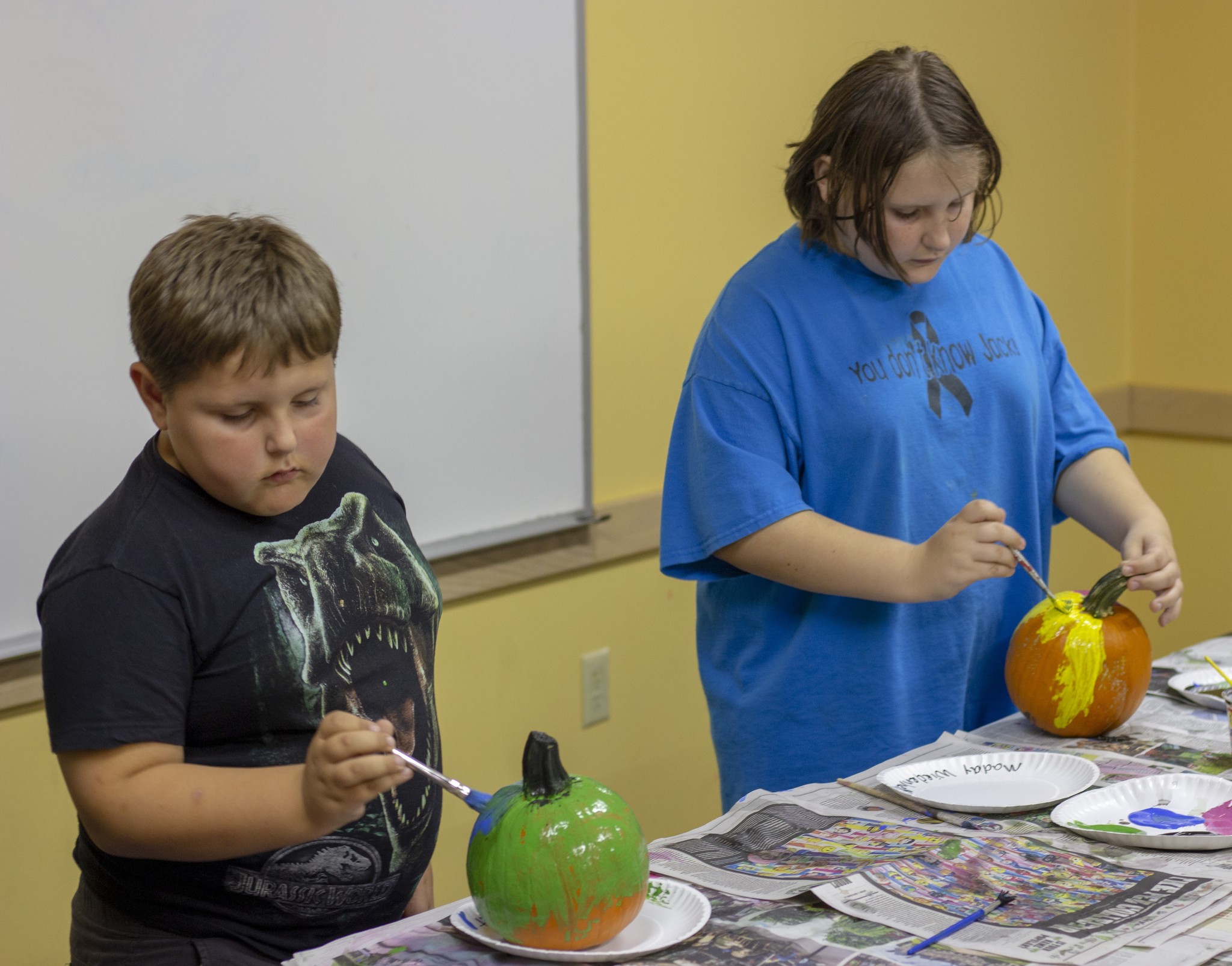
left=656, top=46, right=1187, bottom=819
left=35, top=213, right=450, bottom=964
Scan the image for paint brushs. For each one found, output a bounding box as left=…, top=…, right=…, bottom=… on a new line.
left=1206, top=655, right=1231, bottom=688
left=389, top=750, right=494, bottom=814
left=907, top=890, right=1018, bottom=957
left=1007, top=546, right=1071, bottom=615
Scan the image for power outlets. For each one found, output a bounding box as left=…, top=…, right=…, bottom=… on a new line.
left=581, top=647, right=611, bottom=729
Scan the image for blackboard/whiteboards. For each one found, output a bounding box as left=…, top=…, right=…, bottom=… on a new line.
left=0, top=1, right=613, bottom=676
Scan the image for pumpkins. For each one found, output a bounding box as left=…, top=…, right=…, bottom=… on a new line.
left=1005, top=565, right=1152, bottom=738
left=466, top=730, right=650, bottom=952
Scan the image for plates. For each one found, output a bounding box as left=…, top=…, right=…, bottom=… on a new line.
left=1169, top=664, right=1232, bottom=710
left=451, top=874, right=710, bottom=962
left=875, top=753, right=1099, bottom=813
left=1050, top=772, right=1232, bottom=851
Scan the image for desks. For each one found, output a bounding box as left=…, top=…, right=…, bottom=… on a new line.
left=276, top=630, right=1232, bottom=966
